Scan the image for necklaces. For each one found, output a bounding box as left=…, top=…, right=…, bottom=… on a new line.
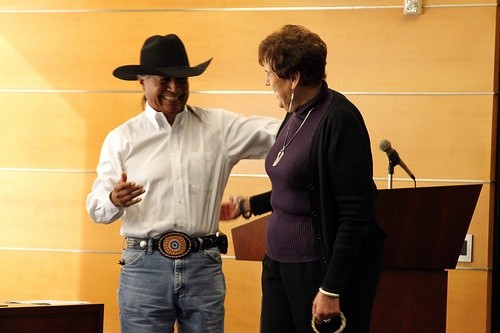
left=272, top=108, right=314, bottom=167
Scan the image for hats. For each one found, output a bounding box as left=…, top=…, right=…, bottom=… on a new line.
left=113, top=34, right=213, bottom=81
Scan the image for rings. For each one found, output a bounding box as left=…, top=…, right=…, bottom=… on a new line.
left=322, top=319, right=332, bottom=323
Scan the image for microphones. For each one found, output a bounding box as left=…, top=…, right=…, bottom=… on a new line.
left=379, top=139, right=415, bottom=180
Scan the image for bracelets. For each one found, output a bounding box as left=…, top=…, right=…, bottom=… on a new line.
left=318, top=287, right=340, bottom=297
left=239, top=197, right=253, bottom=220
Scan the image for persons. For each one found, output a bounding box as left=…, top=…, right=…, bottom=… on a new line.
left=219, top=24, right=386, bottom=333
left=86, top=33, right=283, bottom=333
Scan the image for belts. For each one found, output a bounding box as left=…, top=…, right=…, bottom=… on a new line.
left=126, top=232, right=220, bottom=259
